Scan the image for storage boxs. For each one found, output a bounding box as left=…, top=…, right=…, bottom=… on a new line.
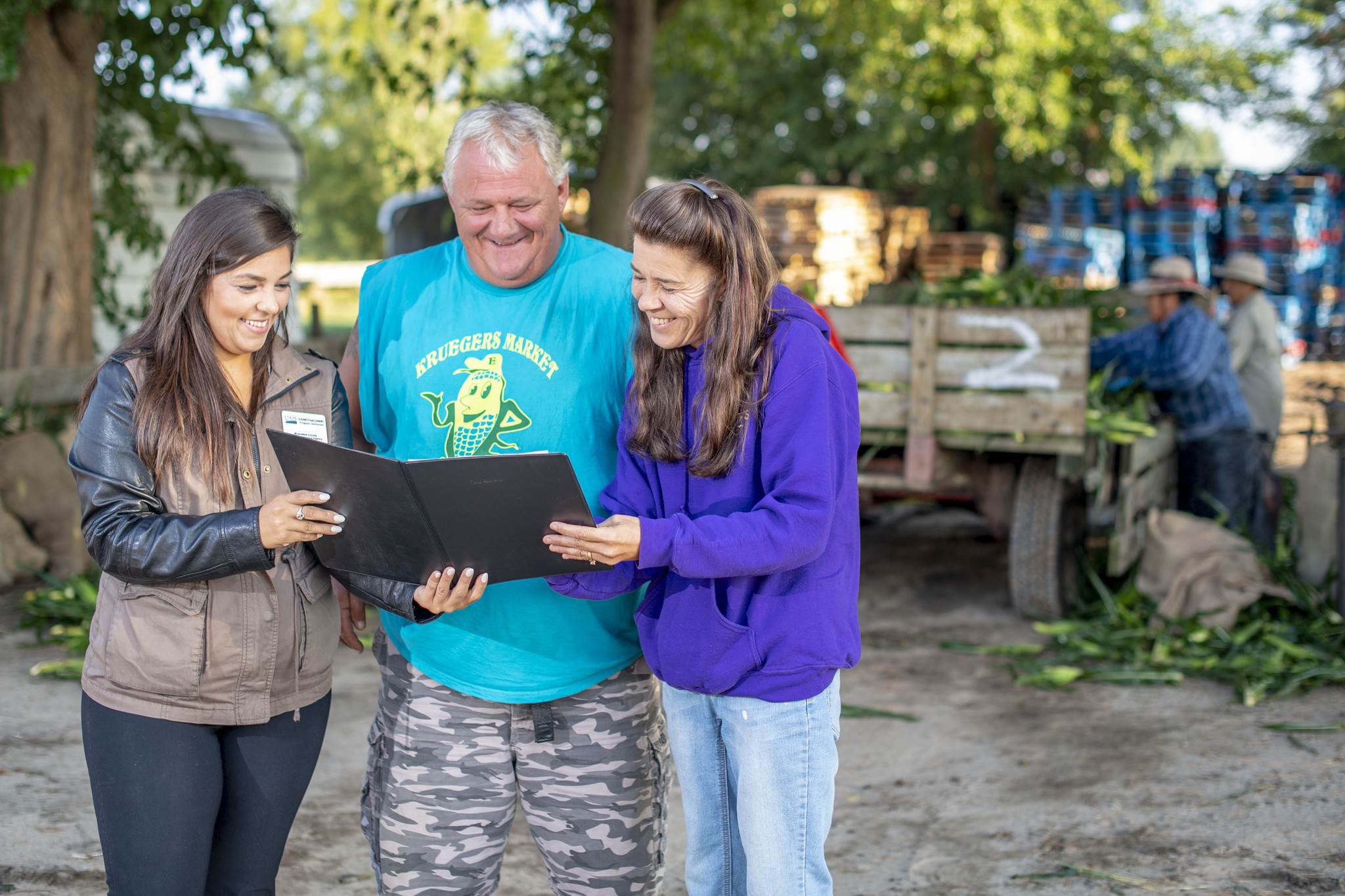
left=750, top=166, right=1345, bottom=363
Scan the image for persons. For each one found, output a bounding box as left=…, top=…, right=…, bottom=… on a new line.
left=1091, top=254, right=1253, bottom=536
left=337, top=100, right=672, bottom=896
left=542, top=178, right=861, bottom=896
left=67, top=191, right=488, bottom=896
left=1209, top=253, right=1284, bottom=552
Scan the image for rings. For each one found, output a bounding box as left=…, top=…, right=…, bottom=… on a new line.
left=588, top=552, right=596, bottom=565
left=296, top=505, right=305, bottom=520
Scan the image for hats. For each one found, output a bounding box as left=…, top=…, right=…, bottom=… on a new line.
left=1210, top=253, right=1282, bottom=292
left=1133, top=255, right=1213, bottom=298
